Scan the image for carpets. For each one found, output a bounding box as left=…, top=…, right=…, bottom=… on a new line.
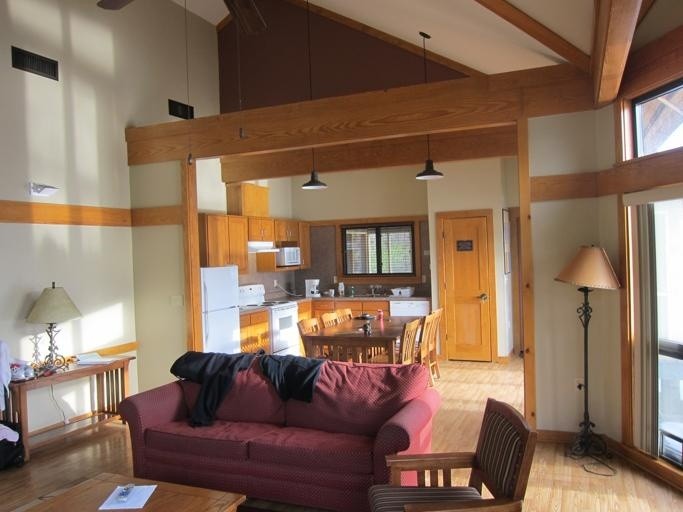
left=237, top=505, right=278, bottom=512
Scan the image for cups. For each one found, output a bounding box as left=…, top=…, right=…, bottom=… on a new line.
left=329, top=289, right=334, bottom=297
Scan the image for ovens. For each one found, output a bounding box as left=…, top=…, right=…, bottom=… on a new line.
left=266, top=305, right=301, bottom=358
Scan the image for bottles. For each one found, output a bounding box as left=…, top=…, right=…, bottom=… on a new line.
left=349, top=285, right=355, bottom=298
left=377, top=310, right=383, bottom=320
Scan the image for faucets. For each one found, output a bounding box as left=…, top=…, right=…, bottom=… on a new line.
left=368, top=284, right=383, bottom=296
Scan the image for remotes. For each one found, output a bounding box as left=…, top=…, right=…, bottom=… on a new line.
left=118, top=483, right=134, bottom=501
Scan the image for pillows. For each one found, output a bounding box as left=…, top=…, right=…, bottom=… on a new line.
left=183, top=356, right=285, bottom=423
left=285, top=363, right=429, bottom=435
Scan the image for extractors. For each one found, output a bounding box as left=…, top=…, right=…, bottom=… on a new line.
left=247, top=241, right=280, bottom=254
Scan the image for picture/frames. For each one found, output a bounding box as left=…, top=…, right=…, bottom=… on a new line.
left=502, top=208, right=512, bottom=275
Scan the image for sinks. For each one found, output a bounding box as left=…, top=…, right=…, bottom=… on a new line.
left=355, top=294, right=387, bottom=297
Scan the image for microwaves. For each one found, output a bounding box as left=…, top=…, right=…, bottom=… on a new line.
left=276, top=247, right=301, bottom=267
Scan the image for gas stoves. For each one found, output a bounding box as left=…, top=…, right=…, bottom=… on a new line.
left=240, top=301, right=297, bottom=310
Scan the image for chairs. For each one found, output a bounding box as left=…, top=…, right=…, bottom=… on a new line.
left=297, top=308, right=353, bottom=361
left=366, top=397, right=538, bottom=512
left=365, top=307, right=444, bottom=387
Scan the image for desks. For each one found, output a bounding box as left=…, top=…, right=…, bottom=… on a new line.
left=12, top=471, right=247, bottom=512
left=6, top=353, right=137, bottom=461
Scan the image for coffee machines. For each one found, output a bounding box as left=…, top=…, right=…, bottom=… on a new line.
left=305, top=279, right=320, bottom=298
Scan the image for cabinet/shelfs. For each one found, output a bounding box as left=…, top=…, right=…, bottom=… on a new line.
left=298, top=300, right=390, bottom=319
left=197, top=182, right=311, bottom=274
left=240, top=308, right=270, bottom=354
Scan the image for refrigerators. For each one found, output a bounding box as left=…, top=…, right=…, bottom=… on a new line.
left=201, top=266, right=241, bottom=355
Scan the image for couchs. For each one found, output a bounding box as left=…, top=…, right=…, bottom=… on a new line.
left=117, top=354, right=443, bottom=512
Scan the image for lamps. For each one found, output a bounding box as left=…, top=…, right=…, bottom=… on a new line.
left=301, top=0, right=328, bottom=190
left=184, top=0, right=192, bottom=166
left=25, top=281, right=82, bottom=370
left=416, top=31, right=446, bottom=180
left=554, top=243, right=621, bottom=456
left=236, top=0, right=244, bottom=138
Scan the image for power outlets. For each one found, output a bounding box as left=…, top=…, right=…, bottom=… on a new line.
left=575, top=377, right=586, bottom=392
left=274, top=280, right=278, bottom=288
